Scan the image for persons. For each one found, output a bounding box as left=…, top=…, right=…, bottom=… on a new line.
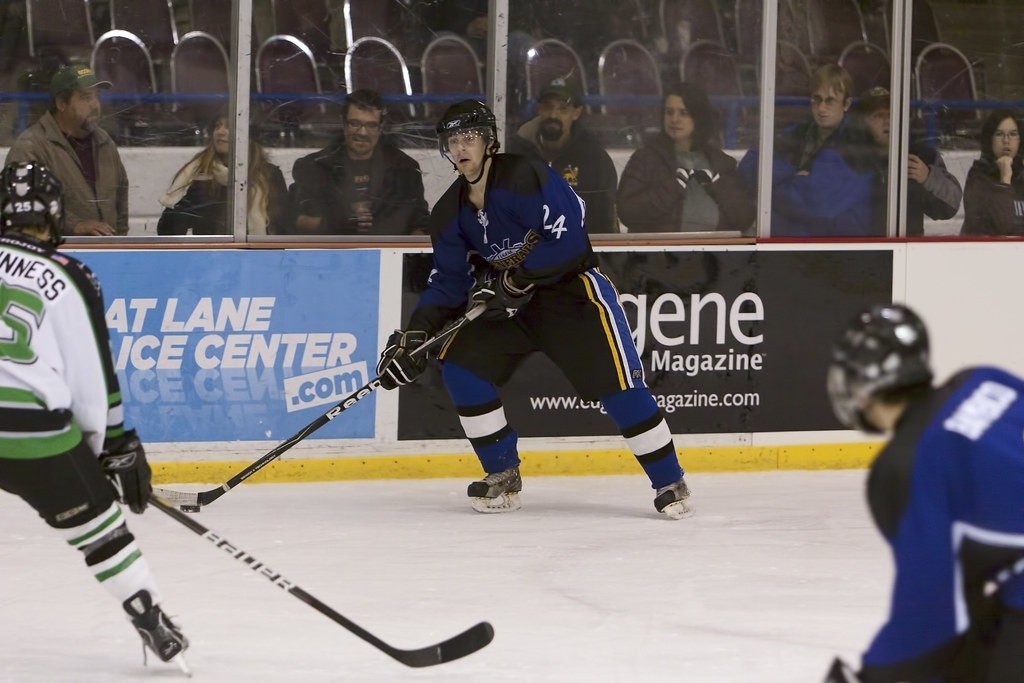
left=740, top=71, right=881, bottom=238
left=853, top=86, right=961, bottom=236
left=508, top=76, right=622, bottom=234
left=960, top=107, right=1024, bottom=237
left=617, top=81, right=740, bottom=232
left=5, top=63, right=129, bottom=236
left=0, top=161, right=190, bottom=663
left=289, top=88, right=431, bottom=237
left=157, top=101, right=289, bottom=235
left=373, top=99, right=690, bottom=512
left=824, top=301, right=1024, bottom=683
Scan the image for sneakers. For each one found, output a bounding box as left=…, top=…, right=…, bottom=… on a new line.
left=468, top=466, right=523, bottom=514
left=122, top=590, right=194, bottom=678
left=654, top=477, right=693, bottom=519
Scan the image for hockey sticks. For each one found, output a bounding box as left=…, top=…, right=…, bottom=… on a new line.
left=147, top=494, right=494, bottom=668
left=152, top=304, right=488, bottom=505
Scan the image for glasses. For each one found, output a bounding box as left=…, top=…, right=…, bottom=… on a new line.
left=810, top=97, right=838, bottom=107
left=343, top=119, right=380, bottom=133
left=993, top=130, right=1020, bottom=141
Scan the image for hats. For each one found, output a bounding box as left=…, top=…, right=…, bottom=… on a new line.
left=50, top=64, right=112, bottom=98
left=860, top=86, right=889, bottom=107
left=536, top=71, right=578, bottom=104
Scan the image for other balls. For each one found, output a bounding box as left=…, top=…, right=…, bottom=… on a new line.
left=180, top=505, right=200, bottom=514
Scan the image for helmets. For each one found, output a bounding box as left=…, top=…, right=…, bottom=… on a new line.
left=0, top=161, right=69, bottom=248
left=437, top=99, right=497, bottom=149
left=826, top=305, right=932, bottom=426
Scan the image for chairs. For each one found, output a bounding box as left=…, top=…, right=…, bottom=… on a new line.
left=1, top=0, right=982, bottom=153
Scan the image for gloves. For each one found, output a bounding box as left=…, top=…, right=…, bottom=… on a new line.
left=465, top=266, right=537, bottom=321
left=694, top=169, right=722, bottom=204
left=375, top=329, right=431, bottom=391
left=670, top=167, right=694, bottom=203
left=96, top=428, right=153, bottom=515
left=825, top=659, right=861, bottom=683
